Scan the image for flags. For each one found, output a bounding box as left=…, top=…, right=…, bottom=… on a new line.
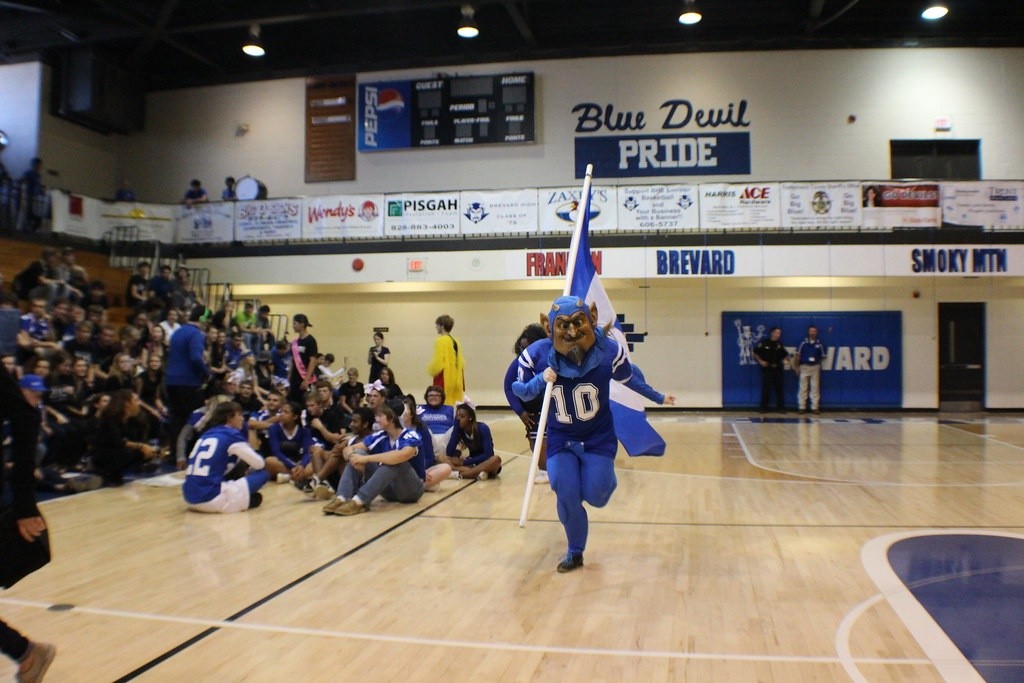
left=519, top=163, right=667, bottom=456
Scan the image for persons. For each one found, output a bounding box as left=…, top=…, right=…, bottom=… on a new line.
left=753, top=327, right=791, bottom=414
left=323, top=400, right=426, bottom=516
left=222, top=176, right=237, bottom=201
left=19, top=158, right=42, bottom=187
left=184, top=179, right=209, bottom=209
left=181, top=402, right=269, bottom=514
left=512, top=295, right=676, bottom=573
left=862, top=185, right=882, bottom=207
left=116, top=179, right=136, bottom=202
left=368, top=332, right=390, bottom=384
left=504, top=323, right=548, bottom=471
left=0, top=246, right=502, bottom=683
left=796, top=326, right=827, bottom=415
left=428, top=315, right=466, bottom=414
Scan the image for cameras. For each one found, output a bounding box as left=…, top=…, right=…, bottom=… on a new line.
left=370, top=347, right=377, bottom=355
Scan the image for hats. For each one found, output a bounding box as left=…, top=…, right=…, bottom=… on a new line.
left=294, top=313, right=314, bottom=329
left=21, top=373, right=47, bottom=393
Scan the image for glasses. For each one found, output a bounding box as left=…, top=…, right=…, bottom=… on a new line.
left=427, top=393, right=442, bottom=398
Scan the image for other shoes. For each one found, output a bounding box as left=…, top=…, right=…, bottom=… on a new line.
left=533, top=470, right=549, bottom=483
left=314, top=479, right=336, bottom=499
left=302, top=473, right=323, bottom=492
left=275, top=472, right=292, bottom=483
left=323, top=498, right=345, bottom=516
left=800, top=409, right=804, bottom=414
left=557, top=553, right=583, bottom=572
left=249, top=492, right=263, bottom=508
left=476, top=471, right=487, bottom=481
left=15, top=643, right=56, bottom=683
left=331, top=500, right=371, bottom=515
left=814, top=410, right=819, bottom=414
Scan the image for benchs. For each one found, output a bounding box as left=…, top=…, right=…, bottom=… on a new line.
left=0, top=236, right=134, bottom=336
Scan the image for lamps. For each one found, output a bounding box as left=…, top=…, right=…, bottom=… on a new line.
left=242, top=22, right=266, bottom=58
left=457, top=2, right=480, bottom=38
left=679, top=0, right=703, bottom=24
left=921, top=0, right=949, bottom=19
